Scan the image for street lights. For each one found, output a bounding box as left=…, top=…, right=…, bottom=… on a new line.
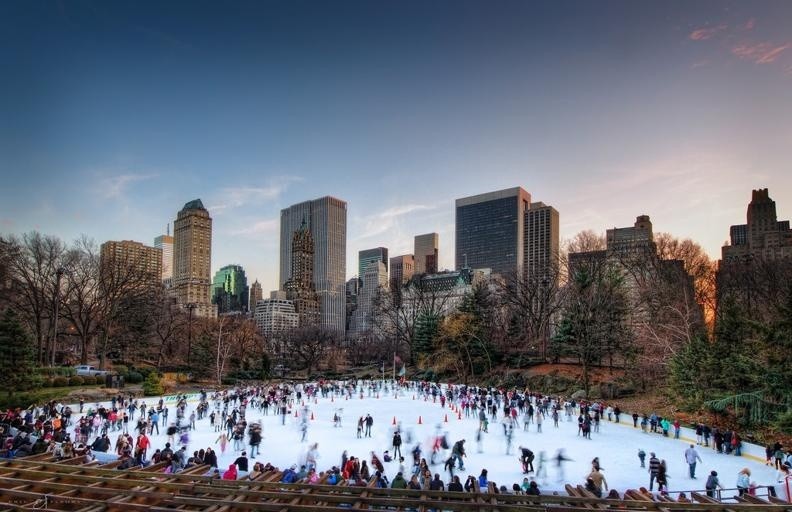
left=185, top=300, right=199, bottom=371
left=742, top=250, right=755, bottom=352
left=50, top=267, right=67, bottom=367
left=395, top=305, right=402, bottom=359
left=541, top=276, right=549, bottom=366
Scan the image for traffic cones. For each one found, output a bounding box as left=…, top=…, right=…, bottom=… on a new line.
left=391, top=415, right=397, bottom=425
left=417, top=416, right=424, bottom=424
left=286, top=396, right=319, bottom=421
left=441, top=401, right=463, bottom=421
left=329, top=391, right=437, bottom=404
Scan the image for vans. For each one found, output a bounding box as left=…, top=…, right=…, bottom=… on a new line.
left=42, top=350, right=70, bottom=363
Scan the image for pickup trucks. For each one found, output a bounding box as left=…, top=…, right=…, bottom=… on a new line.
left=76, top=364, right=110, bottom=378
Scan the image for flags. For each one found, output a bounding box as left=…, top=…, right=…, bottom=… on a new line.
left=394, top=355, right=401, bottom=361
left=397, top=365, right=405, bottom=376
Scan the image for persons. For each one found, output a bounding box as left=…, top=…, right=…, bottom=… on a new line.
left=0, top=378, right=791, bottom=503
